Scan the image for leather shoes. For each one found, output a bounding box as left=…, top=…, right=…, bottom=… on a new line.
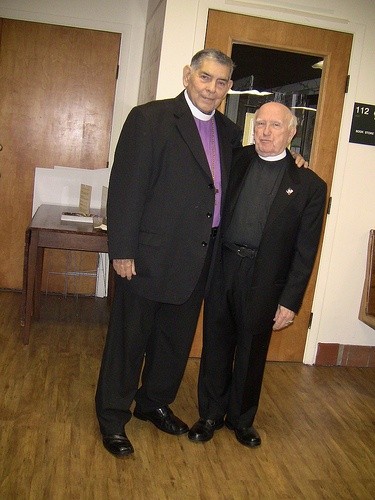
left=188, top=414, right=224, bottom=441
left=103, top=431, right=134, bottom=456
left=133, top=404, right=189, bottom=435
left=225, top=415, right=261, bottom=447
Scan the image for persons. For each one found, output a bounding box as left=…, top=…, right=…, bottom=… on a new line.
left=94, top=49, right=308, bottom=457
left=188, top=102, right=327, bottom=448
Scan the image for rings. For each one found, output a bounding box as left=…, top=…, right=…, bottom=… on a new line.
left=286, top=322, right=289, bottom=324
left=289, top=321, right=293, bottom=325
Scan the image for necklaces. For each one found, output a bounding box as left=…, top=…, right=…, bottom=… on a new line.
left=209, top=116, right=219, bottom=193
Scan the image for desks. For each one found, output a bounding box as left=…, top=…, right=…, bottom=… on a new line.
left=21, top=204, right=109, bottom=345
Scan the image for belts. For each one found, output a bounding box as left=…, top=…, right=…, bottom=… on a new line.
left=210, top=228, right=218, bottom=238
left=223, top=241, right=257, bottom=258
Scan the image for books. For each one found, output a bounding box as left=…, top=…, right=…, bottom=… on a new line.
left=61, top=211, right=94, bottom=223
